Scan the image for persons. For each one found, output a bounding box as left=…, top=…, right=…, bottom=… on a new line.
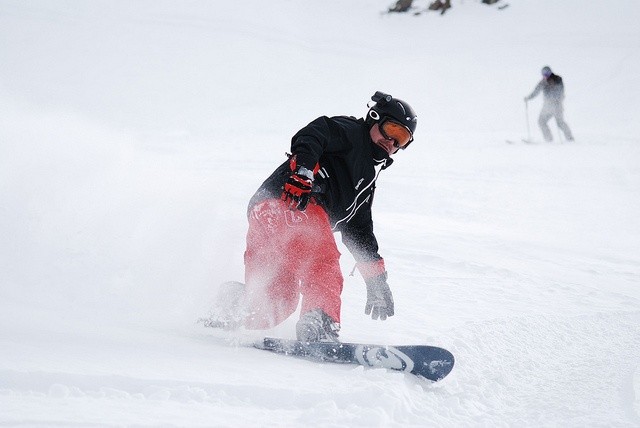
left=525, top=66, right=575, bottom=146
left=197, top=91, right=417, bottom=345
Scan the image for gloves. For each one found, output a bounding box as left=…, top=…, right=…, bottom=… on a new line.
left=283, top=152, right=323, bottom=214
left=364, top=271, right=396, bottom=321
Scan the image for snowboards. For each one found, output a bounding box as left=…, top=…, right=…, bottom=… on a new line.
left=253, top=336, right=455, bottom=385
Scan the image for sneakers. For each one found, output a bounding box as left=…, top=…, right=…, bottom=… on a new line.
left=294, top=308, right=340, bottom=343
left=206, top=279, right=246, bottom=329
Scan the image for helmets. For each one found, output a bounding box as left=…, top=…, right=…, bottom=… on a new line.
left=367, top=91, right=418, bottom=137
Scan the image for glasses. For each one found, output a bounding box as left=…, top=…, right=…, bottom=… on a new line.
left=379, top=112, right=414, bottom=151
